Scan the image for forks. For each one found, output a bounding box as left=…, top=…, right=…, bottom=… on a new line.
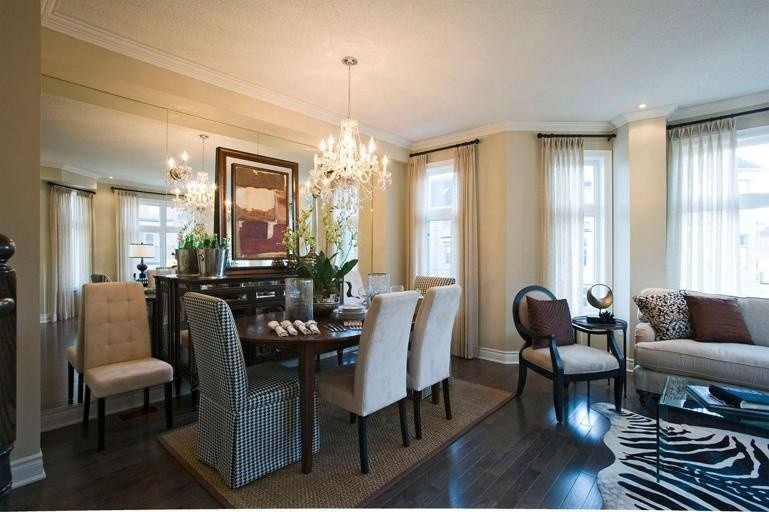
left=342, top=319, right=363, bottom=330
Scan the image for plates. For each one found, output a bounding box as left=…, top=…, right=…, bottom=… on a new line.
left=331, top=304, right=368, bottom=322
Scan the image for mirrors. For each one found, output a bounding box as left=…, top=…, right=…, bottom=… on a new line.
left=41, top=75, right=374, bottom=417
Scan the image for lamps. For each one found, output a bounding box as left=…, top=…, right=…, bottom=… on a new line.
left=304, top=53, right=392, bottom=220
left=128, top=240, right=155, bottom=279
left=183, top=135, right=214, bottom=224
left=168, top=157, right=176, bottom=179
left=175, top=149, right=192, bottom=179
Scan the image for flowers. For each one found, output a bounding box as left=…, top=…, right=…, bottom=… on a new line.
left=276, top=206, right=362, bottom=301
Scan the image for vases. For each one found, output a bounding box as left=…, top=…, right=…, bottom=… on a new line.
left=313, top=301, right=335, bottom=320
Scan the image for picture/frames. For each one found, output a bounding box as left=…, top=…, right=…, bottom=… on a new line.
left=214, top=145, right=300, bottom=278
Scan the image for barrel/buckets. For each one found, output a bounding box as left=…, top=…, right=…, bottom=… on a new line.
left=175, top=249, right=198, bottom=274
left=196, top=248, right=229, bottom=277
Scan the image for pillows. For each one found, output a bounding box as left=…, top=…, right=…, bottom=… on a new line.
left=526, top=296, right=574, bottom=350
left=633, top=290, right=755, bottom=346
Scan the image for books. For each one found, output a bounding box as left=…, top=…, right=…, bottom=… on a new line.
left=684, top=383, right=769, bottom=420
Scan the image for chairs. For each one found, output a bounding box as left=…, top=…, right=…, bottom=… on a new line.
left=511, top=285, right=624, bottom=423
left=80, top=282, right=174, bottom=451
left=66, top=285, right=84, bottom=404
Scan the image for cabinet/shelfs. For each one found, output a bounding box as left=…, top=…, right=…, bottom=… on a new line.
left=153, top=275, right=287, bottom=429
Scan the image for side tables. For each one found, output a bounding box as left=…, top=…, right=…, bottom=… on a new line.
left=571, top=315, right=627, bottom=398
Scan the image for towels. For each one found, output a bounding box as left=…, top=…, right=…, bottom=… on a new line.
left=268, top=320, right=321, bottom=337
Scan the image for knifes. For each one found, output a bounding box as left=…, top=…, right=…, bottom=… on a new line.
left=316, top=318, right=347, bottom=332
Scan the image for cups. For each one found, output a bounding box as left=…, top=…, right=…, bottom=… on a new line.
left=367, top=273, right=391, bottom=304
left=284, top=278, right=312, bottom=321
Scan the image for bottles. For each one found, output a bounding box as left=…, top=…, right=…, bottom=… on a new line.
left=177, top=232, right=228, bottom=249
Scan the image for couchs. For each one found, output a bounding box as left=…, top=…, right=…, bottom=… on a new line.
left=633, top=284, right=769, bottom=408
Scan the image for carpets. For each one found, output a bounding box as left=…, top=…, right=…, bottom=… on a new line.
left=588, top=402, right=769, bottom=512
left=153, top=377, right=517, bottom=508
